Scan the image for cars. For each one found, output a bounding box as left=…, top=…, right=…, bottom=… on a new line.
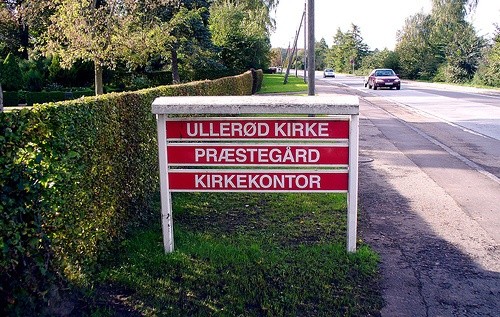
left=368, top=68, right=401, bottom=90
left=322, top=69, right=335, bottom=78
